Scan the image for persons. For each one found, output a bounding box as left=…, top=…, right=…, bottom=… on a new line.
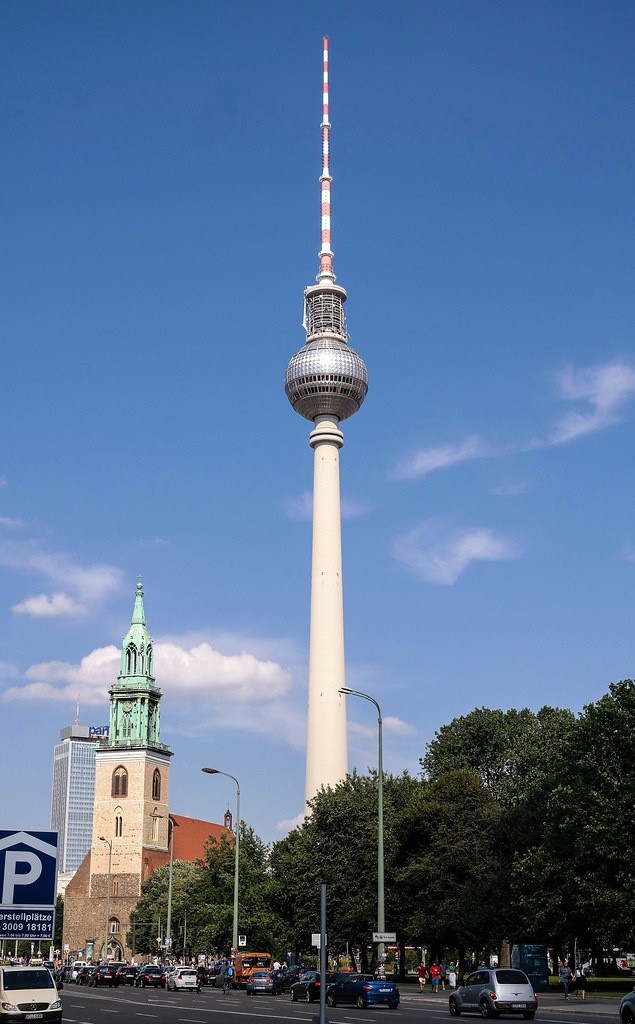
left=197, top=964, right=206, bottom=987
left=333, top=957, right=340, bottom=972
left=447, top=962, right=456, bottom=989
left=477, top=961, right=488, bottom=978
left=222, top=966, right=233, bottom=988
left=271, top=960, right=281, bottom=970
left=560, top=961, right=587, bottom=1000
left=415, top=962, right=428, bottom=994
left=430, top=960, right=440, bottom=993
left=440, top=960, right=447, bottom=991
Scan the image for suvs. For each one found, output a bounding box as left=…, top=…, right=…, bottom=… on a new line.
left=0, top=965, right=64, bottom=1024
left=448, top=965, right=539, bottom=1020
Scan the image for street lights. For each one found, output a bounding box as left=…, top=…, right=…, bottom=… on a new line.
left=99, top=835, right=113, bottom=962
left=337, top=684, right=387, bottom=977
left=202, top=765, right=241, bottom=954
left=150, top=812, right=176, bottom=956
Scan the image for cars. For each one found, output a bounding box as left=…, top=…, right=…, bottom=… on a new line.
left=61, top=952, right=401, bottom=1009
left=619, top=986, right=635, bottom=1024
